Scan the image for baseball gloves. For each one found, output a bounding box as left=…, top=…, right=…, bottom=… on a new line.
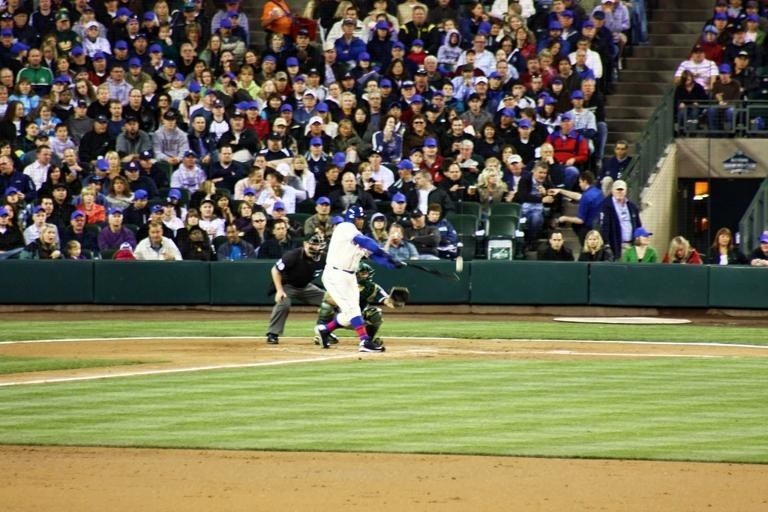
left=389, top=288, right=409, bottom=308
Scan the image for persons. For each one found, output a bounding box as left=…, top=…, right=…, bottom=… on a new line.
left=662, top=236, right=703, bottom=266
left=314, top=262, right=409, bottom=345
left=2, top=1, right=643, bottom=263
left=673, top=1, right=768, bottom=140
left=623, top=227, right=658, bottom=264
left=314, top=205, right=403, bottom=353
left=265, top=231, right=327, bottom=344
left=702, top=227, right=749, bottom=265
left=750, top=231, right=768, bottom=266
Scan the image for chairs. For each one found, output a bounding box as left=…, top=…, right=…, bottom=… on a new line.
left=67, top=159, right=524, bottom=259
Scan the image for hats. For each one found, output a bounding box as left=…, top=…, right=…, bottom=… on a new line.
left=507, top=154, right=522, bottom=165
left=759, top=231, right=768, bottom=243
left=243, top=187, right=256, bottom=195
left=330, top=215, right=344, bottom=225
left=182, top=2, right=238, bottom=28
left=315, top=196, right=331, bottom=205
left=690, top=0, right=760, bottom=73
left=548, top=10, right=605, bottom=30
left=0, top=182, right=85, bottom=218
left=474, top=71, right=584, bottom=129
left=54, top=5, right=178, bottom=60
left=423, top=138, right=437, bottom=147
left=88, top=150, right=182, bottom=215
left=183, top=149, right=197, bottom=156
left=73, top=99, right=86, bottom=107
left=0, top=11, right=30, bottom=54
left=633, top=227, right=653, bottom=239
left=94, top=115, right=108, bottom=123
left=390, top=159, right=422, bottom=218
left=611, top=179, right=628, bottom=191
left=124, top=55, right=328, bottom=145
left=341, top=18, right=443, bottom=112
left=273, top=201, right=285, bottom=211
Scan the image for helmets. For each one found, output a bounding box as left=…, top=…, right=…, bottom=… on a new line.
left=356, top=261, right=376, bottom=289
left=301, top=232, right=327, bottom=262
left=345, top=205, right=368, bottom=230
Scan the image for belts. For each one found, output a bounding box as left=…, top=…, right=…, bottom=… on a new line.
left=333, top=268, right=354, bottom=274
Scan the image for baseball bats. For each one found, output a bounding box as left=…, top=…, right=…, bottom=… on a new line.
left=407, top=261, right=461, bottom=282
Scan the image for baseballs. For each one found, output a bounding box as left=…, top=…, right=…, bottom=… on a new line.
left=456, top=256, right=464, bottom=273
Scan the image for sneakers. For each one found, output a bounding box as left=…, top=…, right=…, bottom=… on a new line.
left=358, top=339, right=385, bottom=352
left=266, top=335, right=279, bottom=344
left=314, top=324, right=330, bottom=348
left=372, top=337, right=384, bottom=346
left=326, top=334, right=338, bottom=344
